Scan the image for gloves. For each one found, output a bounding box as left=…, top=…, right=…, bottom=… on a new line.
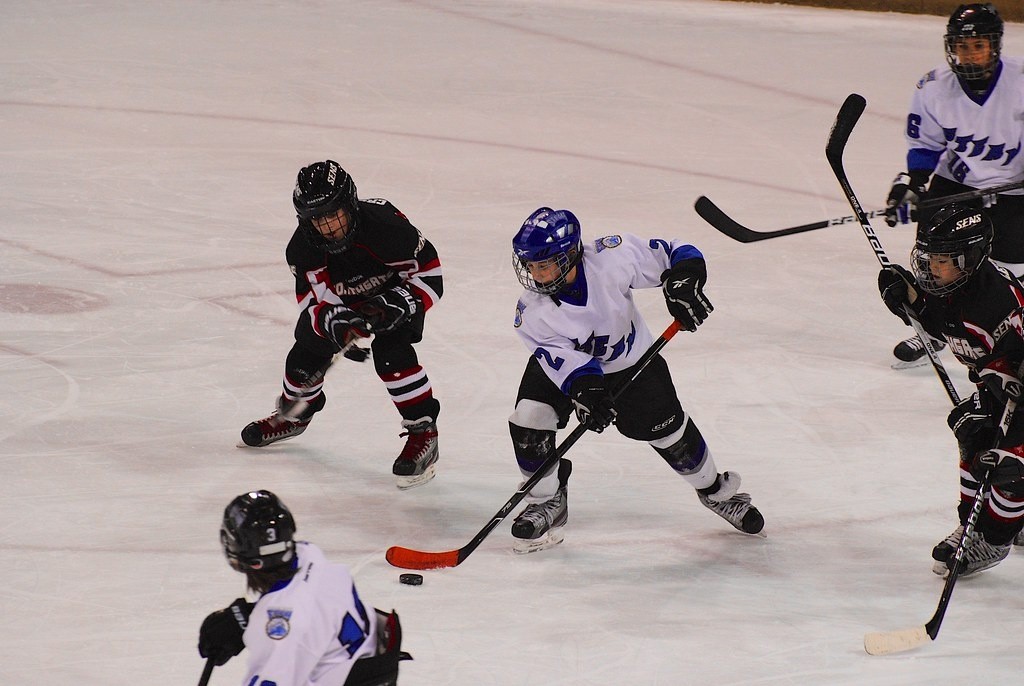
left=879, top=264, right=926, bottom=325
left=569, top=375, right=618, bottom=434
left=947, top=390, right=992, bottom=443
left=318, top=302, right=375, bottom=361
left=661, top=257, right=714, bottom=331
left=885, top=172, right=926, bottom=228
left=360, top=284, right=417, bottom=334
left=198, top=598, right=254, bottom=666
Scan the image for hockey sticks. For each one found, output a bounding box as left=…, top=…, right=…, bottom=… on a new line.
left=823, top=90, right=962, bottom=407
left=272, top=337, right=360, bottom=425
left=694, top=180, right=1024, bottom=245
left=385, top=318, right=686, bottom=570
left=198, top=655, right=215, bottom=686
left=860, top=361, right=1023, bottom=657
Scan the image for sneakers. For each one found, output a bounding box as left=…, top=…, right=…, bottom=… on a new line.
left=393, top=398, right=440, bottom=489
left=235, top=393, right=326, bottom=447
left=891, top=331, right=946, bottom=368
left=695, top=471, right=768, bottom=538
left=933, top=525, right=1013, bottom=579
left=512, top=457, right=572, bottom=553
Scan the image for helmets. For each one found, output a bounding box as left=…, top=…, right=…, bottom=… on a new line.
left=910, top=204, right=993, bottom=296
left=293, top=160, right=359, bottom=254
left=941, top=4, right=1003, bottom=81
left=220, top=490, right=297, bottom=573
left=512, top=206, right=581, bottom=297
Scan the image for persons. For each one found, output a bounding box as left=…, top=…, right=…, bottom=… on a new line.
left=508, top=207, right=764, bottom=539
left=878, top=202, right=1024, bottom=575
left=241, top=159, right=443, bottom=476
left=885, top=2, right=1024, bottom=361
left=198, top=490, right=402, bottom=686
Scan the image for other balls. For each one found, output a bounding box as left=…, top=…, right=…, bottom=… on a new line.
left=399, top=573, right=424, bottom=586
